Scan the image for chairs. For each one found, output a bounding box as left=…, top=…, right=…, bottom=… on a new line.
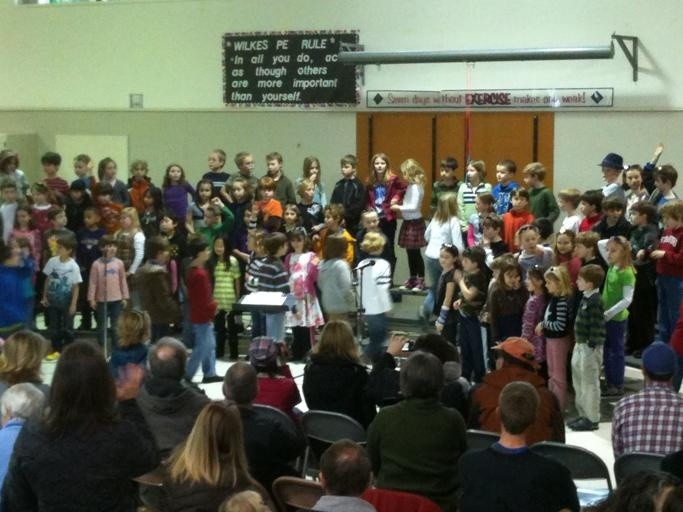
left=245, top=402, right=300, bottom=476
left=301, top=409, right=358, bottom=479
left=362, top=487, right=440, bottom=512
left=464, top=426, right=506, bottom=460
left=615, top=448, right=678, bottom=498
left=273, top=478, right=325, bottom=511
left=527, top=440, right=614, bottom=510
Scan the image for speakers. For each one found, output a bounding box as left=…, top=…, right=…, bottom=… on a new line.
left=292, top=325, right=315, bottom=358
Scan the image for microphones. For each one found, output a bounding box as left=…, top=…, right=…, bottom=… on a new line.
left=353, top=260, right=375, bottom=271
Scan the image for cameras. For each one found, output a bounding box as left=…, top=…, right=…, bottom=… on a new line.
left=401, top=341, right=415, bottom=352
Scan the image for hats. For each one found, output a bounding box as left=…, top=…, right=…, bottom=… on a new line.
left=597, top=153, right=624, bottom=170
left=248, top=336, right=288, bottom=379
left=642, top=341, right=678, bottom=375
left=491, top=336, right=536, bottom=365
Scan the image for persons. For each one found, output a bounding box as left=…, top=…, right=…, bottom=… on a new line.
left=582, top=342, right=682, bottom=511
left=418, top=141, right=683, bottom=431
left=2, top=148, right=426, bottom=382
left=0, top=309, right=581, bottom=512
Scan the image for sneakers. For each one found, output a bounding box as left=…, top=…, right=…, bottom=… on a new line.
left=566, top=416, right=599, bottom=431
